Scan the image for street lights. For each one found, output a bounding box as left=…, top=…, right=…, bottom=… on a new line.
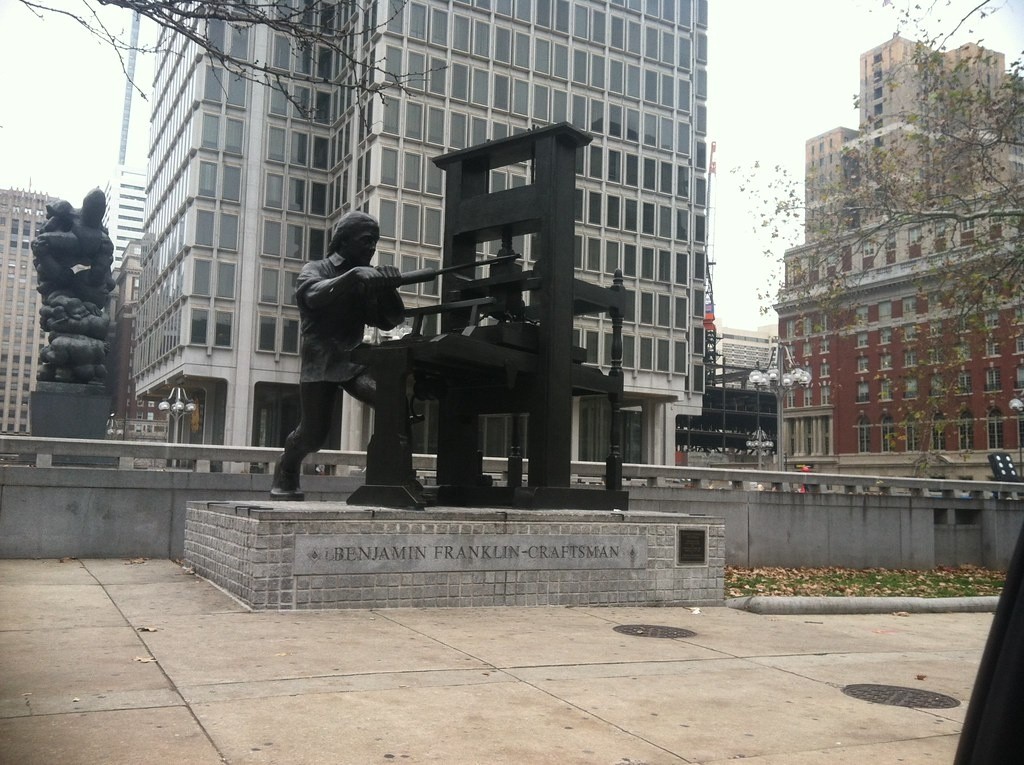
left=749, top=339, right=814, bottom=493
left=744, top=425, right=774, bottom=470
left=1008, top=398, right=1024, bottom=482
left=157, top=386, right=198, bottom=469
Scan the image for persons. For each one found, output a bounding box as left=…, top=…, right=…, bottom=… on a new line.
left=269, top=211, right=425, bottom=508
left=27, top=186, right=118, bottom=325
